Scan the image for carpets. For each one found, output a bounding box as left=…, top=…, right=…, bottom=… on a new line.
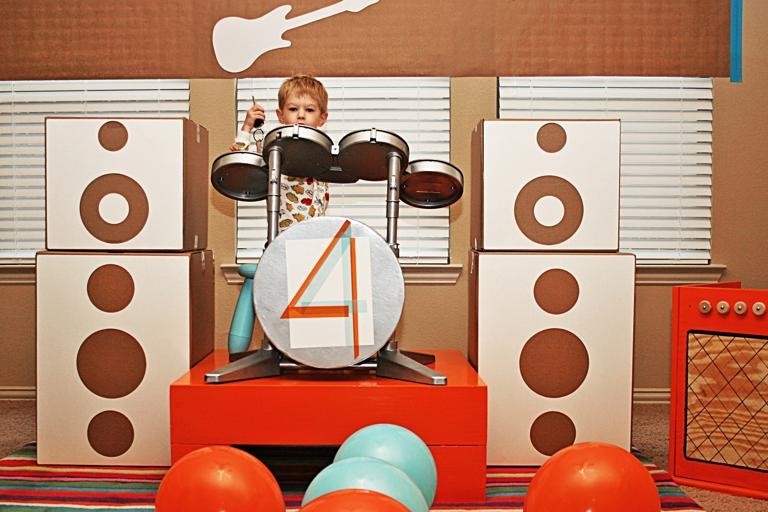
left=0, top=440, right=710, bottom=511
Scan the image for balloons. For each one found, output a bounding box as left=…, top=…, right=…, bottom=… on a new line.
left=523, top=440, right=660, bottom=512
left=155, top=444, right=285, bottom=512
left=300, top=456, right=429, bottom=512
left=298, top=488, right=412, bottom=512
left=333, top=423, right=438, bottom=509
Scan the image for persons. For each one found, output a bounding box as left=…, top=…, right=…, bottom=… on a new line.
left=228, top=70, right=328, bottom=238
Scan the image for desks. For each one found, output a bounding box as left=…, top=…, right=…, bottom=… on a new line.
left=168, top=349, right=488, bottom=505
